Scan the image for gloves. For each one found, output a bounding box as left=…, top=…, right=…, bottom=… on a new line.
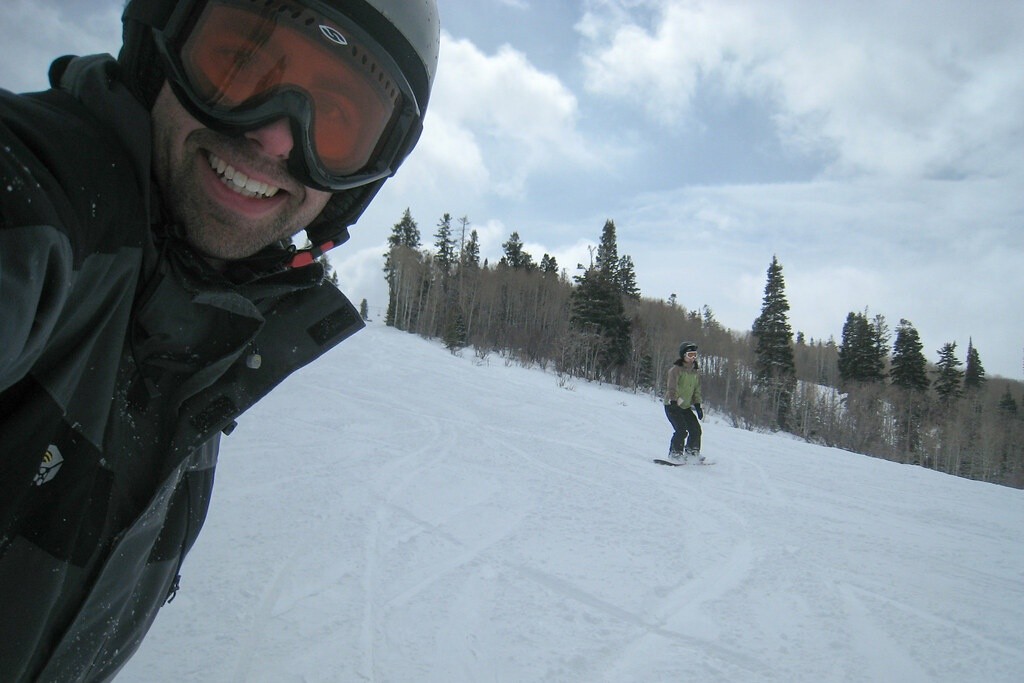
left=694, top=403, right=703, bottom=420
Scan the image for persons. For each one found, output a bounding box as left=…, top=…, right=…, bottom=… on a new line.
left=663, top=341, right=707, bottom=464
left=3, top=1, right=448, bottom=683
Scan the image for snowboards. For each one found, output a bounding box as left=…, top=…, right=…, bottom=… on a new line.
left=654, top=457, right=717, bottom=467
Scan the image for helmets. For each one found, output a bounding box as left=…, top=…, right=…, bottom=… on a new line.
left=122, top=1, right=441, bottom=239
left=679, top=342, right=698, bottom=358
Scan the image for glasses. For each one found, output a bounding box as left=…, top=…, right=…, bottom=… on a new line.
left=684, top=352, right=697, bottom=358
left=121, top=0, right=423, bottom=193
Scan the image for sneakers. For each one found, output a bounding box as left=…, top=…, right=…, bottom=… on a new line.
left=668, top=446, right=687, bottom=462
left=684, top=447, right=706, bottom=462
